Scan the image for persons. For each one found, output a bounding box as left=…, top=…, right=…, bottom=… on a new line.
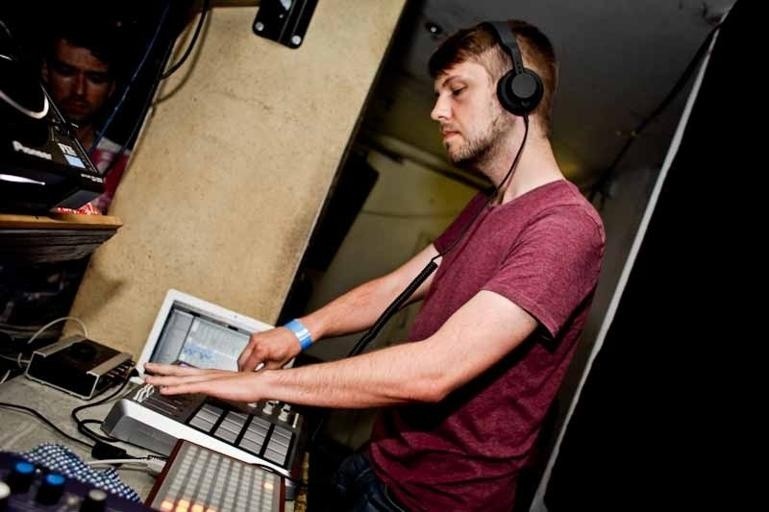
left=139, top=18, right=605, bottom=512
left=37, top=23, right=134, bottom=218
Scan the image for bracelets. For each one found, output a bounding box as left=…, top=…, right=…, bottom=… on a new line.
left=283, top=319, right=312, bottom=353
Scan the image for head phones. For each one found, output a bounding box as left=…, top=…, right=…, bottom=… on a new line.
left=478, top=19, right=544, bottom=116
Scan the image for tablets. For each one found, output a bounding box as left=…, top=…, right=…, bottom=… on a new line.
left=126, top=287, right=297, bottom=403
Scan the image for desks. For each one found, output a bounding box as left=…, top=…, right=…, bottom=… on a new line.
left=1, top=370, right=302, bottom=511
